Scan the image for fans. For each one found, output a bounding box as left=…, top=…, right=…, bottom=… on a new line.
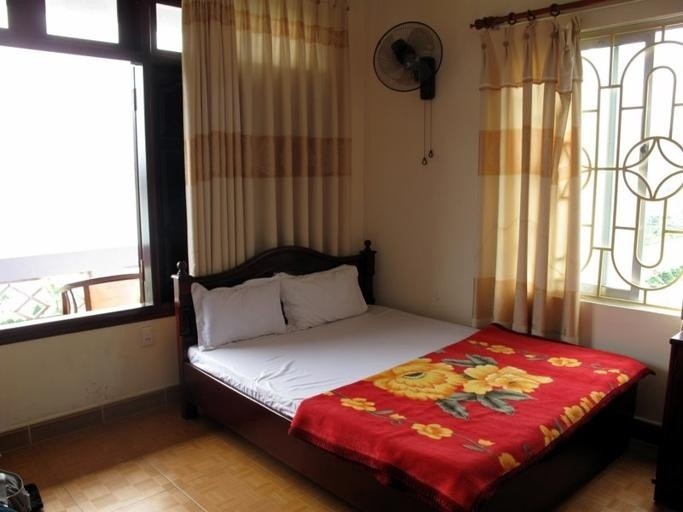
left=372, top=21, right=445, bottom=166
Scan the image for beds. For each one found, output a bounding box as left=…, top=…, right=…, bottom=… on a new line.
left=170, top=239, right=654, bottom=511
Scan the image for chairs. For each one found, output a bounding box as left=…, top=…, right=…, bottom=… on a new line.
left=62, top=273, right=141, bottom=317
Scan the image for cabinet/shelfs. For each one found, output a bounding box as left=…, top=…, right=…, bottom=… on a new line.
left=651, top=333, right=683, bottom=510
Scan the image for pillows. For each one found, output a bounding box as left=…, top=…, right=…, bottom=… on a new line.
left=190, top=275, right=288, bottom=351
left=274, top=266, right=369, bottom=332
